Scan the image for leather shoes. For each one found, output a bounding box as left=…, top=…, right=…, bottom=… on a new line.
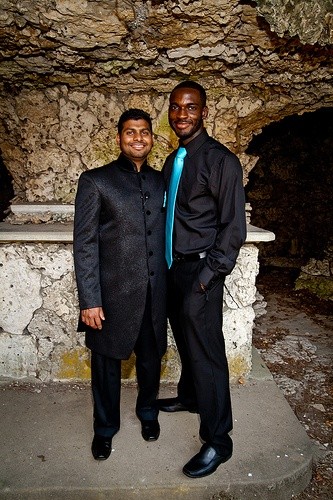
left=158, top=397, right=200, bottom=414
left=91, top=436, right=112, bottom=460
left=141, top=420, right=160, bottom=441
left=182, top=443, right=232, bottom=478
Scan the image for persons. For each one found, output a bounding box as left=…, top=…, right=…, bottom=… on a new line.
left=72, top=109, right=166, bottom=461
left=158, top=80, right=247, bottom=479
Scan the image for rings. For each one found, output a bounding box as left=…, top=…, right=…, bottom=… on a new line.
left=84, top=321, right=89, bottom=323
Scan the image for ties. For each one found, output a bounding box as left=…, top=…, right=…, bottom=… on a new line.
left=165, top=147, right=186, bottom=269
